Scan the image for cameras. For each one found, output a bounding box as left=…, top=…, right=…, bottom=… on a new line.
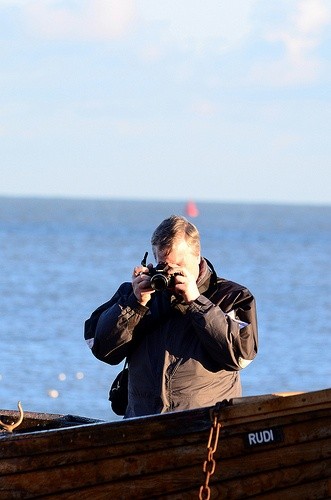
left=141, top=263, right=176, bottom=292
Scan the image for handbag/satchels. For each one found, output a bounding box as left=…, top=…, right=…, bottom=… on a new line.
left=108, top=368, right=128, bottom=416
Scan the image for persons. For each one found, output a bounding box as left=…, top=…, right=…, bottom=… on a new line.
left=83, top=215, right=259, bottom=420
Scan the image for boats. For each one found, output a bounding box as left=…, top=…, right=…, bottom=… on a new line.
left=1, top=389, right=331, bottom=499
